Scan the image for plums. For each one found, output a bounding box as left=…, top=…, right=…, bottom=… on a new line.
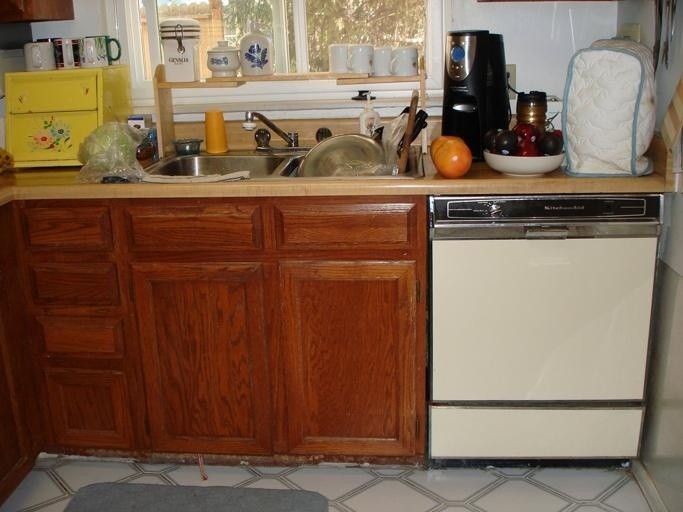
left=485, top=123, right=564, bottom=156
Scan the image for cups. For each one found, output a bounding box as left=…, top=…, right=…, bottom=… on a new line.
left=328, top=43, right=419, bottom=79
left=25, top=36, right=121, bottom=70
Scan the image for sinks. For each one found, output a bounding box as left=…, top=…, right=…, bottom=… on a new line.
left=146, top=152, right=287, bottom=183
left=277, top=148, right=421, bottom=179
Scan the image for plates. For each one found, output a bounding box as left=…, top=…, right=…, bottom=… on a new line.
left=485, top=150, right=566, bottom=179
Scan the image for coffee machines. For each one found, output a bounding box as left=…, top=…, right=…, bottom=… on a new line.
left=441, top=30, right=510, bottom=158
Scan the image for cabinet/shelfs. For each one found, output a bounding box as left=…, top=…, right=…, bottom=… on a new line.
left=3, top=64, right=133, bottom=169
left=125, top=195, right=428, bottom=465
left=11, top=198, right=136, bottom=458
left=0, top=202, right=40, bottom=507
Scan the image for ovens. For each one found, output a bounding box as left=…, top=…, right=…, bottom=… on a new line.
left=431, top=196, right=669, bottom=469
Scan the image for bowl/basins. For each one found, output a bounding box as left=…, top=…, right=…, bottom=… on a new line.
left=173, top=140, right=201, bottom=154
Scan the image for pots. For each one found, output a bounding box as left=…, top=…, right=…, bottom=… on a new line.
left=297, top=106, right=413, bottom=176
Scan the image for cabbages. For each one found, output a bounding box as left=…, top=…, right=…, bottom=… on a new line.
left=77, top=123, right=136, bottom=171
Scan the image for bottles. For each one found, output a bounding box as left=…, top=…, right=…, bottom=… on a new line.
left=205, top=20, right=272, bottom=77
left=517, top=91, right=547, bottom=134
left=204, top=109, right=228, bottom=154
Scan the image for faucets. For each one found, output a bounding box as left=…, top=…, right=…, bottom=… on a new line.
left=240, top=109, right=299, bottom=148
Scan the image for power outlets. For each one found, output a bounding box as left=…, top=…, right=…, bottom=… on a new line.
left=505, top=64, right=517, bottom=100
